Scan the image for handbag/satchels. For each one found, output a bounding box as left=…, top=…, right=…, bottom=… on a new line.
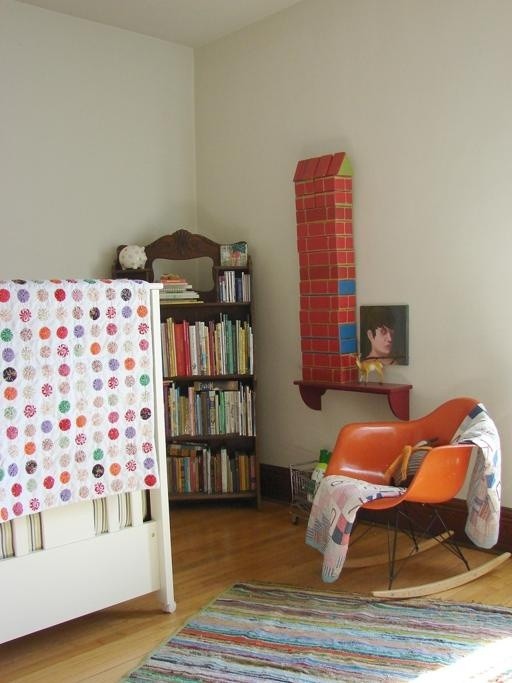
left=385, top=440, right=437, bottom=488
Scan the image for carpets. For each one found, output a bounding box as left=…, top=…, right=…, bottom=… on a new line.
left=116, top=581, right=512, bottom=683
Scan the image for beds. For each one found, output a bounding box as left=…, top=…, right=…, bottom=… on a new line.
left=0, top=278, right=178, bottom=648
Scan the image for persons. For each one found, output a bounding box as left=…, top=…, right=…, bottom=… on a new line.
left=361, top=304, right=404, bottom=364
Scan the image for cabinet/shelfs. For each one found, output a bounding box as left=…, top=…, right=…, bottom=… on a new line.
left=110, top=227, right=264, bottom=512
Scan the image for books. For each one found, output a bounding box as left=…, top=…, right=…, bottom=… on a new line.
left=157, top=268, right=258, bottom=496
left=219, top=240, right=247, bottom=267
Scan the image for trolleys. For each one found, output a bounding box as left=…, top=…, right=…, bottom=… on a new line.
left=289, top=459, right=319, bottom=525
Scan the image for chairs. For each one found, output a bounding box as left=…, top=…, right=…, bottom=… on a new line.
left=323, top=396, right=512, bottom=601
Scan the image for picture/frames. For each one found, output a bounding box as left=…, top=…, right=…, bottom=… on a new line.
left=357, top=304, right=412, bottom=363
